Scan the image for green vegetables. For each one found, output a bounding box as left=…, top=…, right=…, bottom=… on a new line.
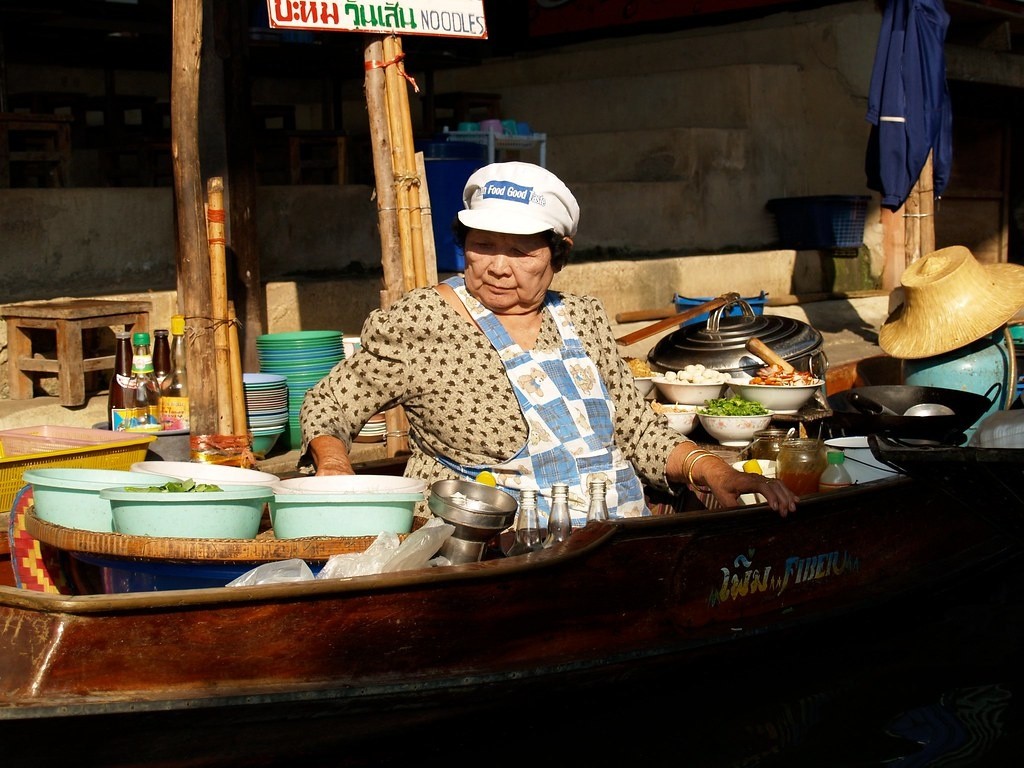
left=698, top=395, right=770, bottom=416
left=123, top=478, right=226, bottom=492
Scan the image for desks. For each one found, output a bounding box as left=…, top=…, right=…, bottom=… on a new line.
left=0, top=112, right=75, bottom=188
left=254, top=129, right=347, bottom=183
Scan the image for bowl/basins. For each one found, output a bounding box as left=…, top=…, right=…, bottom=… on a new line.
left=696, top=410, right=776, bottom=447
left=634, top=371, right=664, bottom=398
left=724, top=376, right=826, bottom=414
left=650, top=376, right=723, bottom=405
left=21, top=460, right=426, bottom=542
left=242, top=330, right=387, bottom=457
left=659, top=404, right=706, bottom=435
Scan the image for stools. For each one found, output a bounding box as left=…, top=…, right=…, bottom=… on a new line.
left=1, top=296, right=154, bottom=408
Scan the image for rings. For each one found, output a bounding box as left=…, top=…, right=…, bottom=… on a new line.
left=766, top=480, right=771, bottom=485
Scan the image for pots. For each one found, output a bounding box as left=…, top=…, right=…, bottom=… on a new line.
left=645, top=300, right=829, bottom=394
left=827, top=381, right=1002, bottom=445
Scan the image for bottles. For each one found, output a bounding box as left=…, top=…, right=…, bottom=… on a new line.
left=751, top=429, right=793, bottom=461
left=775, top=437, right=826, bottom=496
left=543, top=483, right=572, bottom=549
left=474, top=471, right=508, bottom=561
left=819, top=451, right=851, bottom=493
left=107, top=315, right=191, bottom=434
left=585, top=481, right=608, bottom=523
left=506, top=488, right=544, bottom=556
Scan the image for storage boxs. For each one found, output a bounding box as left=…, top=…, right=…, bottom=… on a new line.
left=767, top=195, right=870, bottom=247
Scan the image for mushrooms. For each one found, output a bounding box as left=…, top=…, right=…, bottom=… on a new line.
left=656, top=364, right=730, bottom=384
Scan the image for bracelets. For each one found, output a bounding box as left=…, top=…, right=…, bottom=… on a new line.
left=682, top=449, right=727, bottom=493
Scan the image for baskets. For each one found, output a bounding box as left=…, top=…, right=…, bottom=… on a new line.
left=26, top=507, right=427, bottom=598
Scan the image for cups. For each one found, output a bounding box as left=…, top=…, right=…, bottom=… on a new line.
left=459, top=119, right=532, bottom=142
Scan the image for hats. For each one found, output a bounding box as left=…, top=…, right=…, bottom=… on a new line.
left=457, top=161, right=580, bottom=239
left=878, top=244, right=1024, bottom=360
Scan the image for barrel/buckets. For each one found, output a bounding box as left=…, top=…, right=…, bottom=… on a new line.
left=670, top=291, right=770, bottom=328
left=428, top=478, right=518, bottom=566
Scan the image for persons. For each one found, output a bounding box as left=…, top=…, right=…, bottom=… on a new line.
left=294, top=163, right=799, bottom=536
